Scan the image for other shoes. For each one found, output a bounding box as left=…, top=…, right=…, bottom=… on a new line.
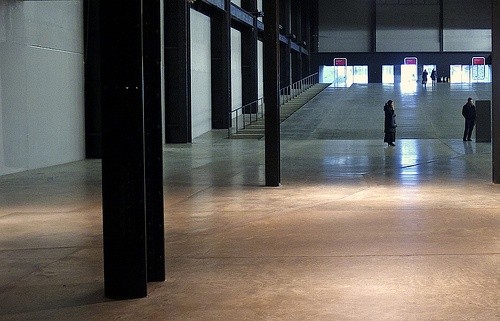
left=467, top=138, right=472, bottom=141
left=463, top=137, right=467, bottom=141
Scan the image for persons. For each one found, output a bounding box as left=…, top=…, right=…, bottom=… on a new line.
left=430, top=68, right=436, bottom=86
left=422, top=69, right=428, bottom=90
left=383, top=100, right=397, bottom=148
left=461, top=97, right=476, bottom=142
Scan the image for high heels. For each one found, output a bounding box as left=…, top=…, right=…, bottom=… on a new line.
left=388, top=142, right=395, bottom=147
